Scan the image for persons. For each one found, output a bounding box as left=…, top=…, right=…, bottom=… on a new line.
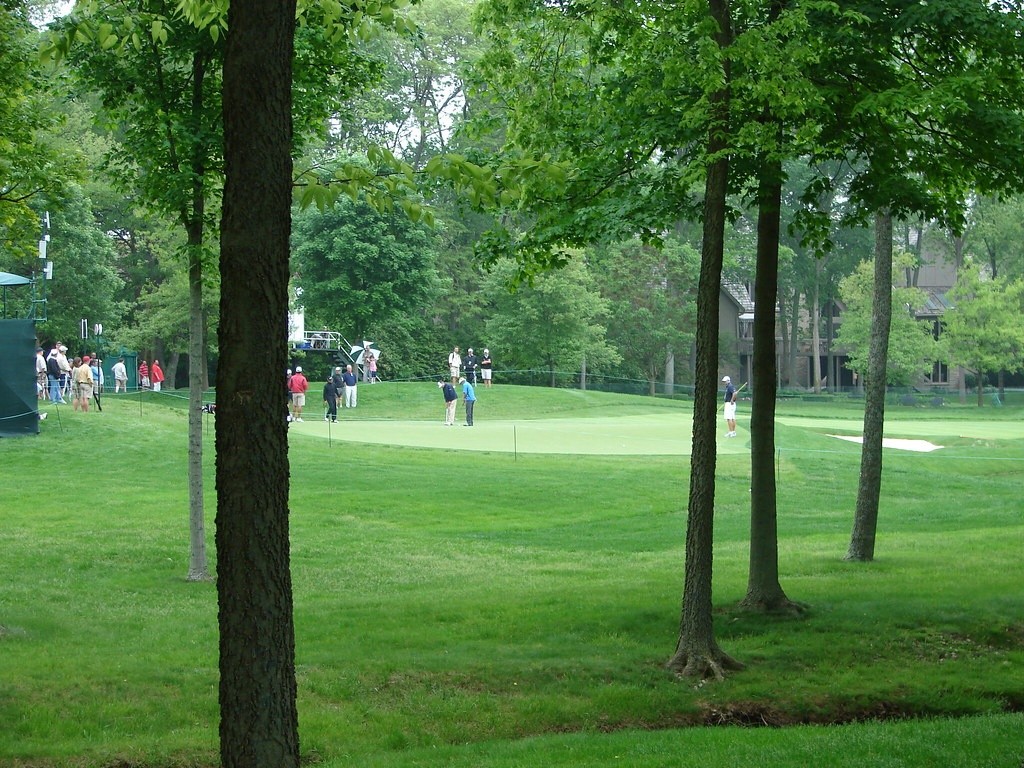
left=152, top=359, right=165, bottom=391
left=458, top=377, right=477, bottom=426
left=481, top=349, right=492, bottom=388
left=721, top=376, right=737, bottom=438
left=111, top=358, right=128, bottom=393
left=369, top=355, right=378, bottom=384
left=448, top=346, right=462, bottom=389
left=286, top=364, right=358, bottom=423
left=438, top=380, right=459, bottom=425
left=139, top=360, right=149, bottom=387
left=463, top=348, right=478, bottom=386
left=363, top=346, right=373, bottom=382
left=33, top=341, right=104, bottom=412
left=319, top=325, right=328, bottom=349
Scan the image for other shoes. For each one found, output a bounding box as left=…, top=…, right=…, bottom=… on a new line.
left=336, top=406, right=342, bottom=409
left=371, top=382, right=375, bottom=384
left=352, top=404, right=356, bottom=407
left=346, top=404, right=350, bottom=408
left=453, top=386, right=455, bottom=388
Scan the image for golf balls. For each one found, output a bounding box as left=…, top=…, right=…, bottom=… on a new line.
left=458, top=424, right=460, bottom=426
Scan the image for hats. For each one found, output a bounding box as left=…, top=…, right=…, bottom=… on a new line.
left=327, top=376, right=333, bottom=380
left=438, top=380, right=443, bottom=388
left=722, top=376, right=730, bottom=381
left=36, top=347, right=45, bottom=351
left=83, top=356, right=92, bottom=362
left=58, top=346, right=68, bottom=351
left=484, top=349, right=489, bottom=352
left=347, top=365, right=351, bottom=368
left=68, top=359, right=74, bottom=362
left=336, top=367, right=341, bottom=370
left=459, top=377, right=466, bottom=384
left=468, top=348, right=473, bottom=352
left=287, top=369, right=292, bottom=374
left=74, top=357, right=81, bottom=362
left=96, top=359, right=102, bottom=362
left=51, top=349, right=58, bottom=354
left=296, top=367, right=302, bottom=372
left=56, top=342, right=62, bottom=345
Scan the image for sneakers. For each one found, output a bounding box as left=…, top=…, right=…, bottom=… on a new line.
left=49, top=401, right=67, bottom=405
left=292, top=417, right=304, bottom=422
left=463, top=423, right=473, bottom=426
left=325, top=417, right=338, bottom=423
left=725, top=431, right=736, bottom=438
left=444, top=421, right=453, bottom=425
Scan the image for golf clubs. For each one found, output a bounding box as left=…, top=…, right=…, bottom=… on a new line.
left=325, top=404, right=327, bottom=421
left=454, top=402, right=465, bottom=421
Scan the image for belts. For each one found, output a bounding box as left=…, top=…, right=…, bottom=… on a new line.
left=39, top=371, right=46, bottom=373
